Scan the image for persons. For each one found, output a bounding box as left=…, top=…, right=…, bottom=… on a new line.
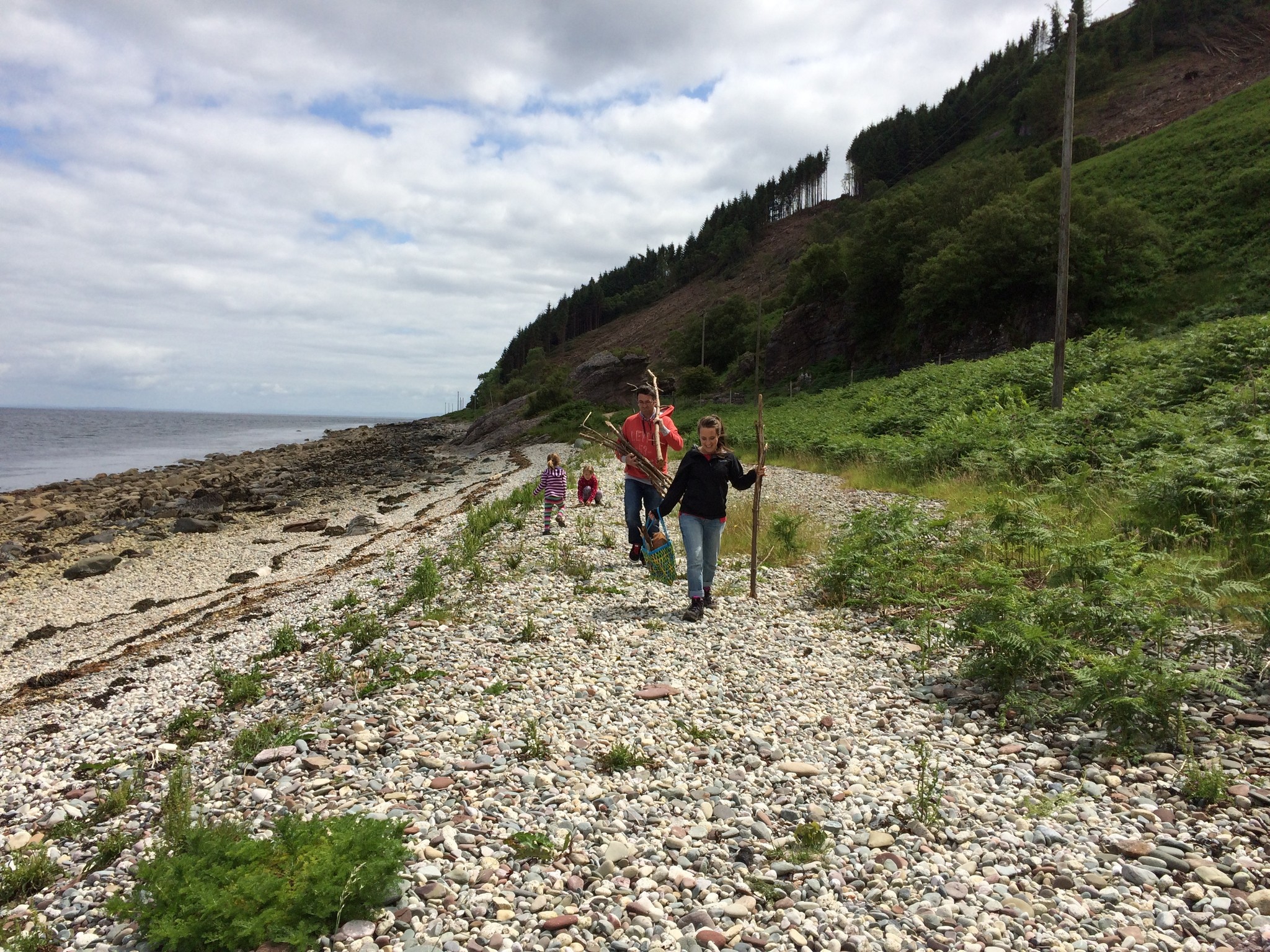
left=532, top=453, right=567, bottom=535
left=615, top=384, right=684, bottom=568
left=648, top=415, right=768, bottom=620
left=577, top=465, right=605, bottom=506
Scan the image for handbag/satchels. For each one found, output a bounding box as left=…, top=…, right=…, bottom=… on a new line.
left=641, top=508, right=676, bottom=586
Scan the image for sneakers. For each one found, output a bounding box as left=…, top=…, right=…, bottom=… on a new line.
left=683, top=596, right=704, bottom=621
left=704, top=589, right=717, bottom=608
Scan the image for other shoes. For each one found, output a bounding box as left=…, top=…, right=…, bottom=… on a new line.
left=543, top=531, right=551, bottom=536
left=595, top=500, right=604, bottom=507
left=555, top=516, right=565, bottom=527
left=629, top=542, right=642, bottom=563
left=640, top=552, right=648, bottom=567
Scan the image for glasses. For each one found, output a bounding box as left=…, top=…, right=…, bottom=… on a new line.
left=637, top=401, right=653, bottom=406
